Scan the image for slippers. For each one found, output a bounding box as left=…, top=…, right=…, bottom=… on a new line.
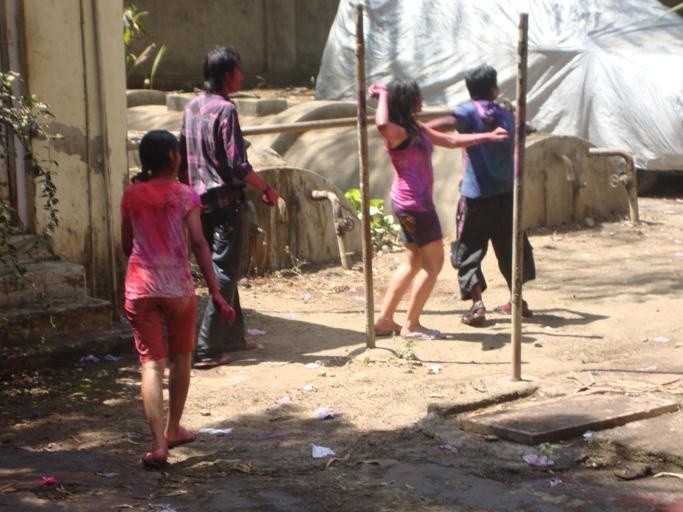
left=143, top=450, right=167, bottom=470
left=399, top=325, right=441, bottom=341
left=193, top=351, right=232, bottom=368
left=375, top=320, right=405, bottom=336
left=163, top=426, right=197, bottom=447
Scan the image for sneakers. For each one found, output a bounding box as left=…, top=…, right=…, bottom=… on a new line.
left=460, top=303, right=487, bottom=325
left=494, top=301, right=532, bottom=317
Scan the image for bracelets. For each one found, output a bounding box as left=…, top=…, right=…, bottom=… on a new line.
left=261, top=185, right=273, bottom=194
left=208, top=286, right=222, bottom=294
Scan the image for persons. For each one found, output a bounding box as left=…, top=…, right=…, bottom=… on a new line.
left=175, top=44, right=280, bottom=371
left=367, top=72, right=509, bottom=343
left=426, top=62, right=537, bottom=328
left=119, top=129, right=237, bottom=465
